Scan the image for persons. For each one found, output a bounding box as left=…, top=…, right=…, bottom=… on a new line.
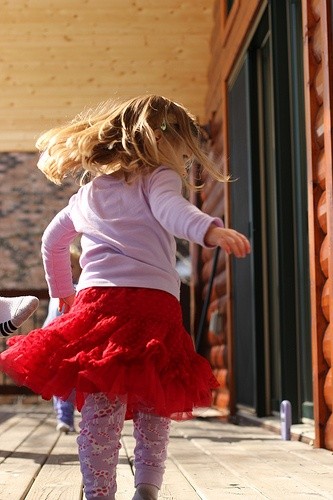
left=0, top=295, right=39, bottom=337
left=38, top=242, right=82, bottom=434
left=0, top=94, right=252, bottom=500
